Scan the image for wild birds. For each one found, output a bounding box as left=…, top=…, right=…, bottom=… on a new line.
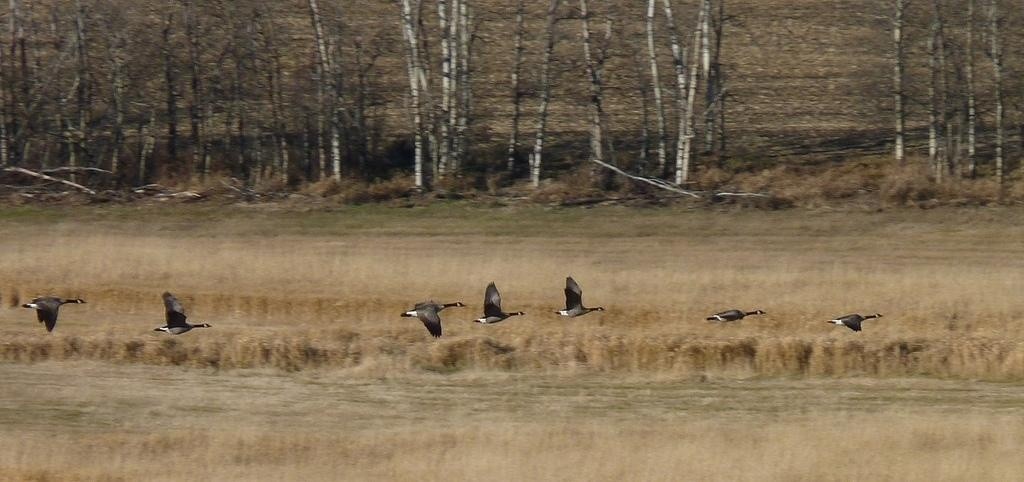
left=706, top=310, right=766, bottom=322
left=21, top=296, right=89, bottom=333
left=474, top=281, right=526, bottom=324
left=827, top=314, right=883, bottom=332
left=154, top=291, right=212, bottom=336
left=400, top=297, right=467, bottom=340
left=554, top=277, right=605, bottom=318
